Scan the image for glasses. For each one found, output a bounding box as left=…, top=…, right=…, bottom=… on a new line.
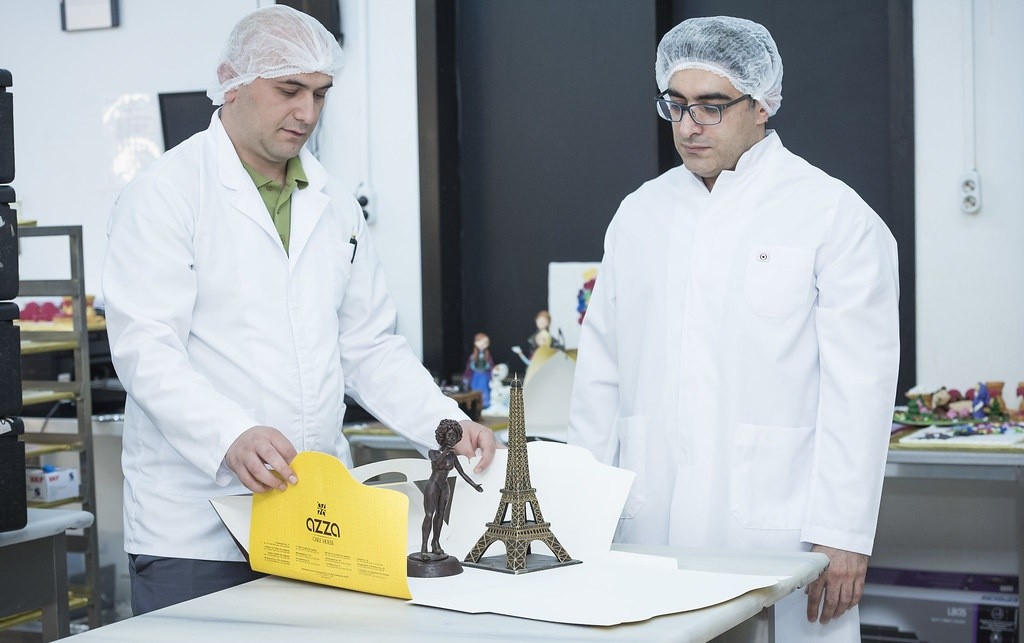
left=654, top=87, right=752, bottom=125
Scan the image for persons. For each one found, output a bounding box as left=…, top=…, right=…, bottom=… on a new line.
left=105, top=4, right=495, bottom=620
left=459, top=312, right=562, bottom=418
left=556, top=14, right=899, bottom=643
left=419, top=419, right=484, bottom=562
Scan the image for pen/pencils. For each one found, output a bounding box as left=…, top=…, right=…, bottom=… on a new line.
left=349, top=233, right=358, bottom=264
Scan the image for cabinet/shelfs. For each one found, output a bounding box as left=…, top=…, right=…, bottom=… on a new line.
left=0, top=223, right=99, bottom=643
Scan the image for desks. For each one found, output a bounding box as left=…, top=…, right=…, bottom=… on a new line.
left=38, top=552, right=830, bottom=643
